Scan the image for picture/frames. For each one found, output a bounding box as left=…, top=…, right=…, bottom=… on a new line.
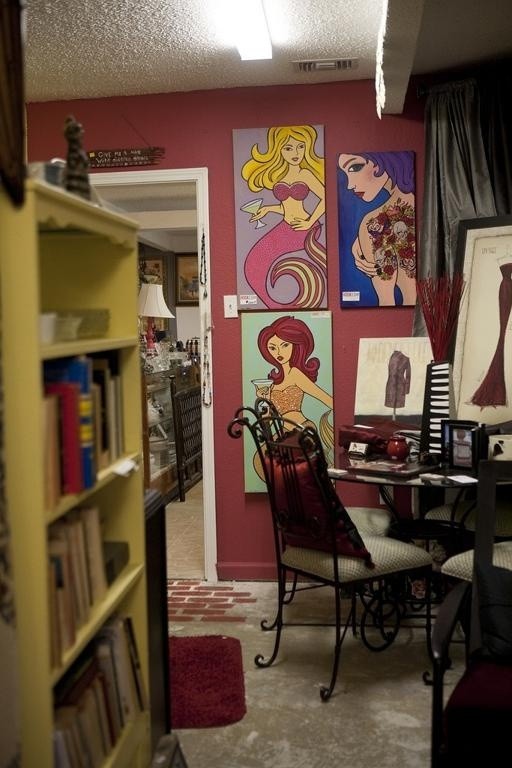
left=449, top=425, right=477, bottom=469
left=441, top=419, right=477, bottom=469
left=175, top=251, right=198, bottom=306
left=453, top=216, right=512, bottom=431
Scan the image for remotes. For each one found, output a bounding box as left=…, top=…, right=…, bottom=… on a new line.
left=418, top=472, right=445, bottom=481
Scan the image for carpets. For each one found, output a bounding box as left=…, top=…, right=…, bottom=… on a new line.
left=169, top=635, right=247, bottom=728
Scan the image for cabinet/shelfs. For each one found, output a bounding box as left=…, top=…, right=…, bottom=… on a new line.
left=142, top=360, right=203, bottom=506
left=1, top=178, right=151, bottom=767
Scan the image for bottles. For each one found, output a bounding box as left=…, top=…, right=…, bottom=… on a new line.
left=386, top=435, right=409, bottom=463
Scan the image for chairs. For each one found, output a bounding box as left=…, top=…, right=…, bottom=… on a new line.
left=423, top=540, right=512, bottom=689
left=234, top=402, right=392, bottom=635
left=230, top=416, right=439, bottom=702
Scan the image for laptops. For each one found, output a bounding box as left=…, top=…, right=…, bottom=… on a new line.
left=346, top=458, right=437, bottom=480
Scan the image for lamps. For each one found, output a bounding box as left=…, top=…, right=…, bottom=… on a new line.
left=138, top=283, right=175, bottom=349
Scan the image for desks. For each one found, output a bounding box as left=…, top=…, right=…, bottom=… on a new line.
left=326, top=446, right=510, bottom=658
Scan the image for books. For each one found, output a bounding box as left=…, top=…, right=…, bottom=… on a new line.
left=53, top=615, right=145, bottom=768
left=48, top=508, right=110, bottom=672
left=45, top=352, right=123, bottom=508
